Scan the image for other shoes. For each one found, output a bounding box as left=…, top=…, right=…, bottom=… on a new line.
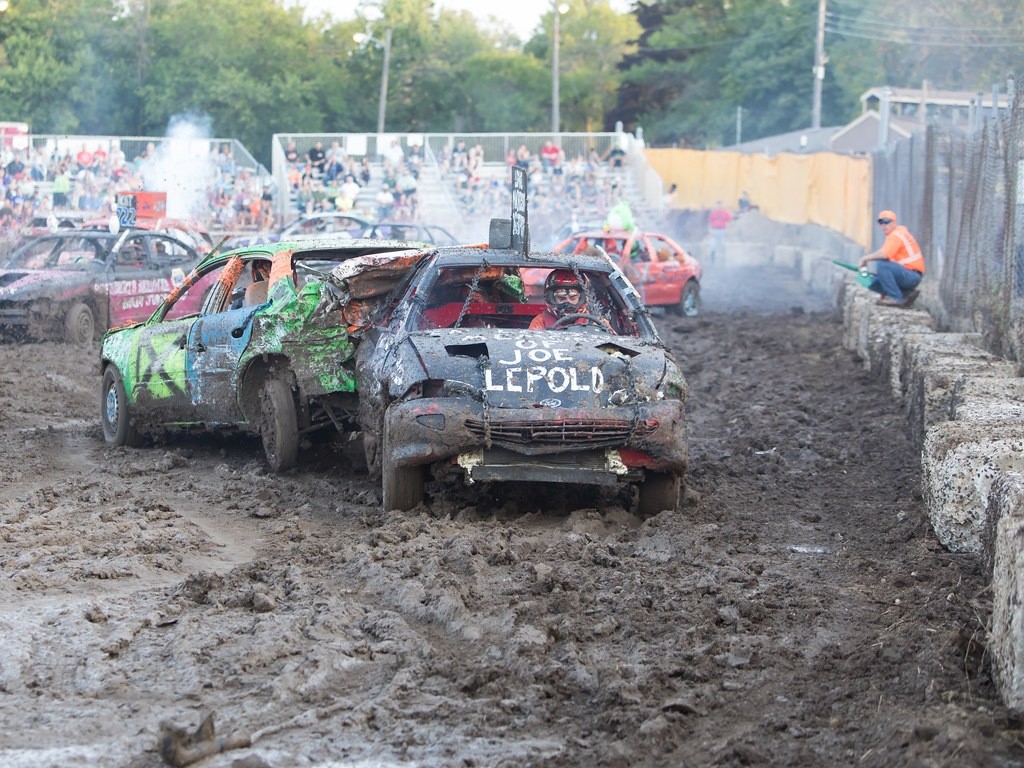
left=904, top=289, right=920, bottom=307
left=876, top=294, right=904, bottom=306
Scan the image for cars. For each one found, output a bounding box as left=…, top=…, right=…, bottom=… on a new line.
left=516, top=225, right=702, bottom=323
left=354, top=246, right=691, bottom=517
left=0, top=208, right=462, bottom=351
left=96, top=236, right=527, bottom=473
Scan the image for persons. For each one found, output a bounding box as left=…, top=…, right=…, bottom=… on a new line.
left=736, top=191, right=751, bottom=210
left=859, top=210, right=925, bottom=307
left=528, top=269, right=618, bottom=336
left=231, top=259, right=297, bottom=309
left=435, top=137, right=629, bottom=239
left=0, top=140, right=273, bottom=229
left=622, top=240, right=643, bottom=263
left=286, top=140, right=424, bottom=221
left=707, top=201, right=732, bottom=264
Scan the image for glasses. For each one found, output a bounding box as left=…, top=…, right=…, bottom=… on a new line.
left=553, top=293, right=581, bottom=300
left=878, top=218, right=892, bottom=224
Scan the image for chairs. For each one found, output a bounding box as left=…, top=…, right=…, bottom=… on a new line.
left=243, top=279, right=270, bottom=305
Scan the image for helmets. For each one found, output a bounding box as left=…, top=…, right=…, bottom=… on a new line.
left=544, top=269, right=586, bottom=323
left=251, top=258, right=297, bottom=287
left=631, top=241, right=639, bottom=257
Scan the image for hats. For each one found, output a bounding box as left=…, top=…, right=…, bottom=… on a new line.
left=878, top=210, right=896, bottom=222
